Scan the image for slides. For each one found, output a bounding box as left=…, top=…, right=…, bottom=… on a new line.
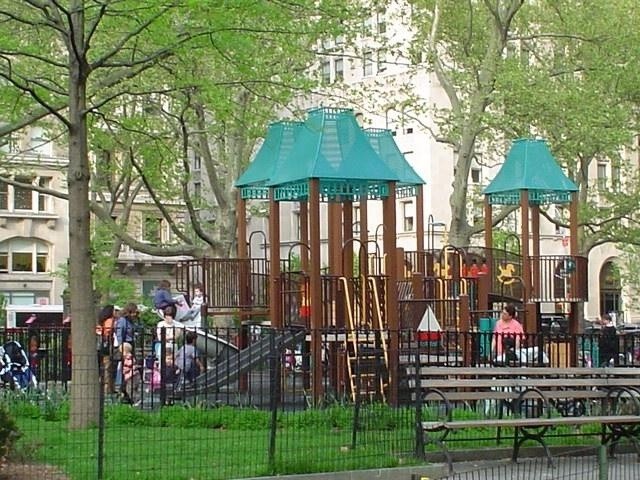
left=152, top=309, right=304, bottom=399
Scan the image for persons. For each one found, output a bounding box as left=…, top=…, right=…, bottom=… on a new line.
left=153, top=280, right=209, bottom=385
left=485, top=306, right=550, bottom=417
left=98, top=303, right=141, bottom=408
left=601, top=314, right=616, bottom=338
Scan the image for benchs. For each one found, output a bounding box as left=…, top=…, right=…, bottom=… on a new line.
left=406, top=361, right=640, bottom=476
